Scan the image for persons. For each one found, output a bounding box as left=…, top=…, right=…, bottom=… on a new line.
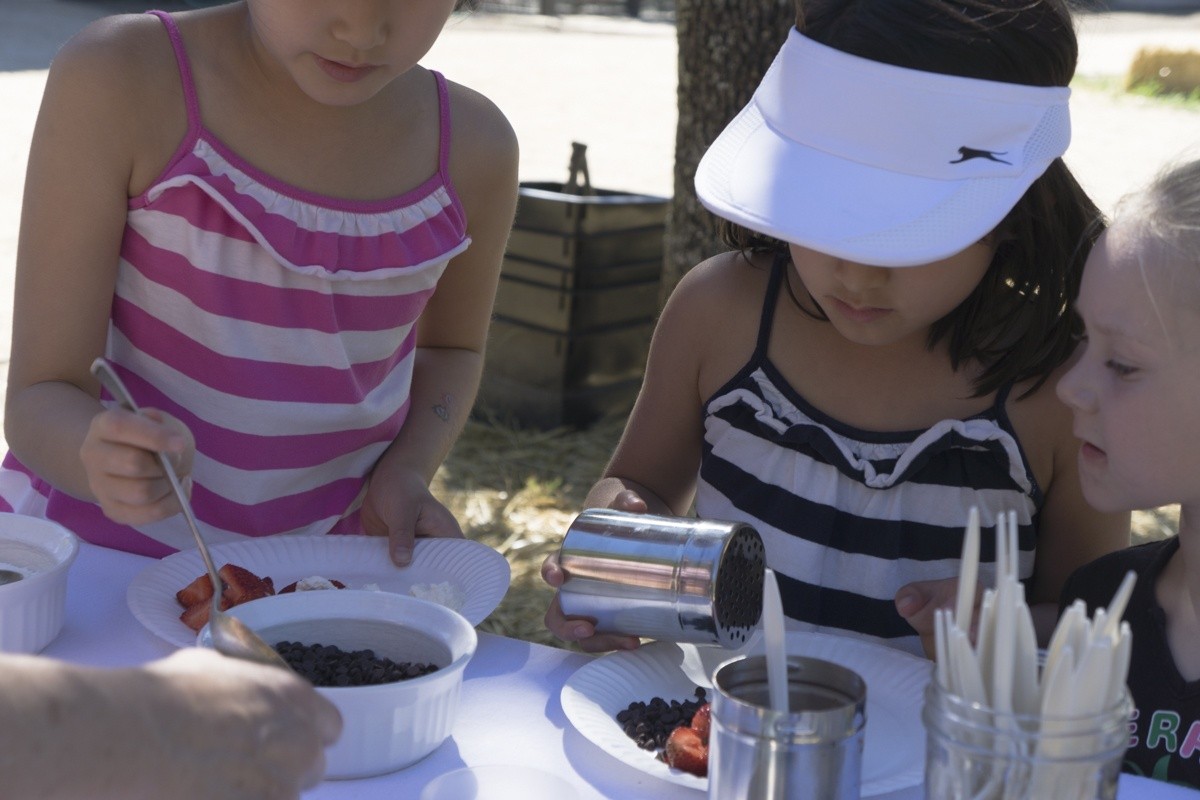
left=1056, top=160, right=1200, bottom=792
left=0, top=0, right=521, bottom=569
left=539, top=0, right=1133, bottom=662
left=0, top=646, right=343, bottom=800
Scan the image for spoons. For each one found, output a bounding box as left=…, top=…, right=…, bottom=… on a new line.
left=91, top=357, right=294, bottom=670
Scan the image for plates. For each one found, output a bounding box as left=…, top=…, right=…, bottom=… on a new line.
left=560, top=632, right=932, bottom=797
left=126, top=534, right=512, bottom=649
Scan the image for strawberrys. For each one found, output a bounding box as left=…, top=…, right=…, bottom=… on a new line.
left=173, top=564, right=276, bottom=632
left=665, top=704, right=711, bottom=776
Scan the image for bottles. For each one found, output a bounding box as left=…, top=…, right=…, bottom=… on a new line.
left=919, top=648, right=1135, bottom=800
left=558, top=506, right=765, bottom=650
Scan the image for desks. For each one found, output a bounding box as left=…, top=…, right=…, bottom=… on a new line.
left=41, top=536, right=1200, bottom=800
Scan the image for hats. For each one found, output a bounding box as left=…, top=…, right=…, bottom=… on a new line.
left=695, top=24, right=1071, bottom=268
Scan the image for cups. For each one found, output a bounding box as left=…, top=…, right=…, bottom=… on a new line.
left=708, top=652, right=866, bottom=800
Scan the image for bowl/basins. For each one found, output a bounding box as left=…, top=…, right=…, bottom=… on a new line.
left=196, top=590, right=477, bottom=780
left=1, top=512, right=80, bottom=654
